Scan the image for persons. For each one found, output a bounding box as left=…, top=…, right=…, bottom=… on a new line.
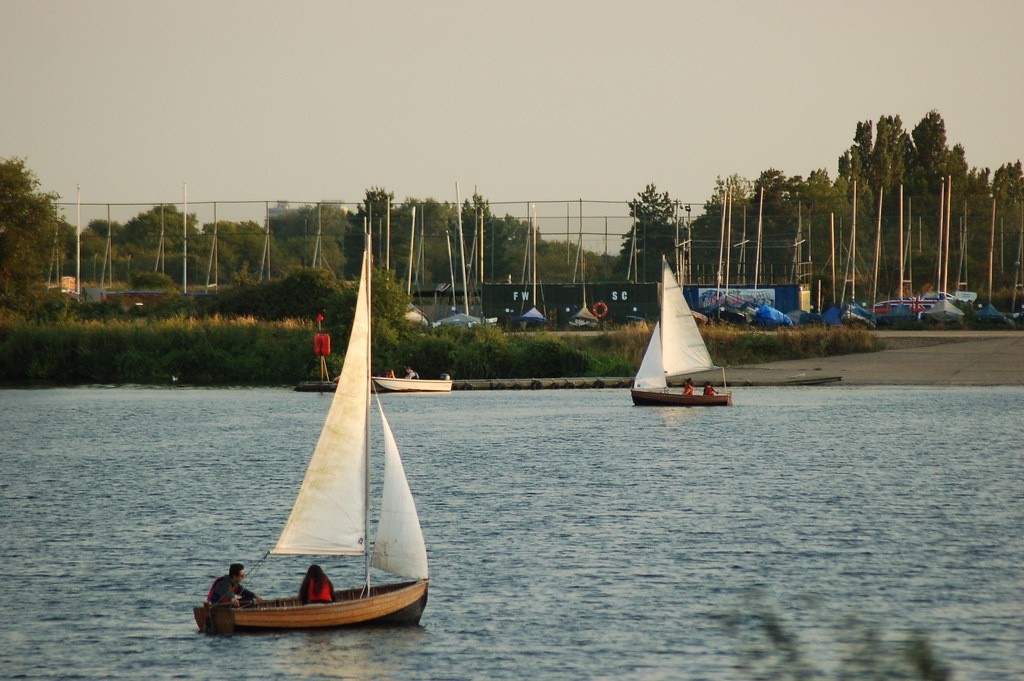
left=703, top=382, right=718, bottom=395
left=386, top=369, right=394, bottom=378
left=520, top=319, right=526, bottom=332
left=684, top=378, right=694, bottom=395
left=208, top=563, right=262, bottom=608
left=300, top=565, right=335, bottom=605
left=404, top=367, right=419, bottom=379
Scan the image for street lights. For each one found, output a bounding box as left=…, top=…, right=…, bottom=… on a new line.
left=487, top=217, right=494, bottom=282
left=685, top=206, right=691, bottom=284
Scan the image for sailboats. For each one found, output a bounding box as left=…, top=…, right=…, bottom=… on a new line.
left=877, top=184, right=917, bottom=326
left=677, top=187, right=823, bottom=329
left=966, top=199, right=1017, bottom=330
left=839, top=180, right=878, bottom=330
left=404, top=181, right=498, bottom=329
left=568, top=232, right=598, bottom=331
left=511, top=213, right=548, bottom=329
left=631, top=254, right=732, bottom=406
left=192, top=233, right=430, bottom=631
left=921, top=176, right=966, bottom=324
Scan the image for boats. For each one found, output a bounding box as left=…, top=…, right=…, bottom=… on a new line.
left=371, top=373, right=453, bottom=393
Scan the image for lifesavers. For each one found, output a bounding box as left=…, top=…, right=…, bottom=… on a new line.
left=592, top=302, right=608, bottom=317
